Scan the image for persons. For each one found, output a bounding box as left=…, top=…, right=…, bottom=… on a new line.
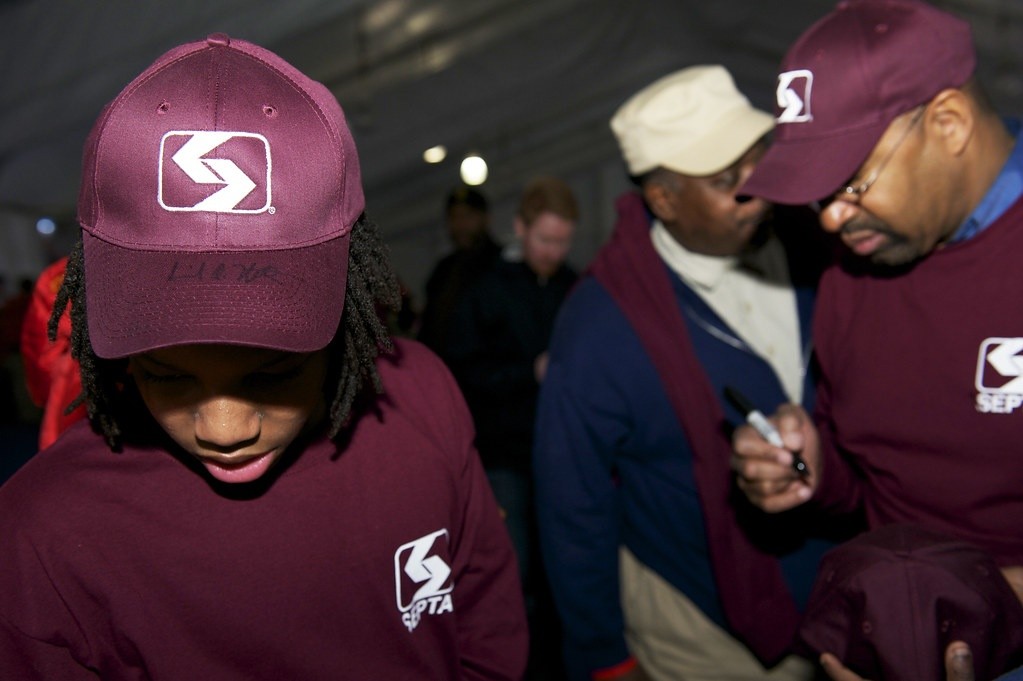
left=726, top=0, right=1023, bottom=681
left=535, top=64, right=853, bottom=681
left=373, top=176, right=581, bottom=681
left=0, top=254, right=89, bottom=454
left=1, top=37, right=527, bottom=681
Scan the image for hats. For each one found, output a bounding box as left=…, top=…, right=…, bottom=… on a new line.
left=739, top=0, right=977, bottom=205
left=606, top=64, right=778, bottom=178
left=79, top=33, right=365, bottom=358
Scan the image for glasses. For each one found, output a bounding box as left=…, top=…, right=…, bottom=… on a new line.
left=836, top=103, right=930, bottom=204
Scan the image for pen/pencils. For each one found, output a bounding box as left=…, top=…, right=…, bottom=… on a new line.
left=723, top=382, right=812, bottom=479
